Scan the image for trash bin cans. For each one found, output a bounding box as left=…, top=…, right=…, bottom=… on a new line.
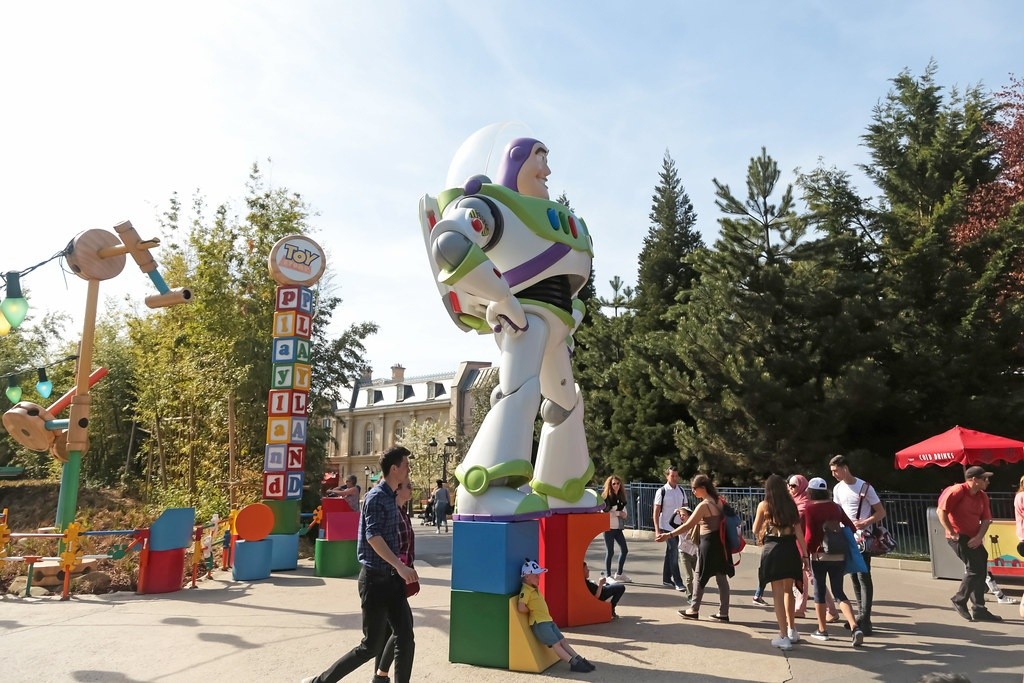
left=925, top=506, right=967, bottom=580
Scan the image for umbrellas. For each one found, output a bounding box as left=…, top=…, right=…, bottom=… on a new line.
left=894, top=425, right=1024, bottom=482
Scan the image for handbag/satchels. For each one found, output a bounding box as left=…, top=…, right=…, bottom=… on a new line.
left=446, top=502, right=455, bottom=515
left=407, top=581, right=420, bottom=597
left=866, top=527, right=897, bottom=556
left=822, top=532, right=848, bottom=554
left=724, top=504, right=742, bottom=547
left=842, top=526, right=867, bottom=575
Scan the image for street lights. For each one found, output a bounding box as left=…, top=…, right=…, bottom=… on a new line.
left=428, top=437, right=458, bottom=488
left=364, top=466, right=370, bottom=495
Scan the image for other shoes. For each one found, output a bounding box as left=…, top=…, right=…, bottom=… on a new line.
left=436, top=530, right=440, bottom=534
left=972, top=610, right=1002, bottom=622
left=998, top=595, right=1020, bottom=605
left=372, top=675, right=390, bottom=683
left=953, top=601, right=971, bottom=621
left=302, top=676, right=318, bottom=683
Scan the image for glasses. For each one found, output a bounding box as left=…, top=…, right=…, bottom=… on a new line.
left=693, top=486, right=699, bottom=490
left=787, top=484, right=797, bottom=488
left=346, top=480, right=351, bottom=483
left=613, top=483, right=619, bottom=485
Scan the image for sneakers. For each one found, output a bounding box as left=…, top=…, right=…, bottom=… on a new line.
left=605, top=576, right=619, bottom=584
left=571, top=662, right=591, bottom=672
left=753, top=597, right=873, bottom=651
left=614, top=572, right=632, bottom=583
left=581, top=658, right=595, bottom=670
left=663, top=582, right=729, bottom=623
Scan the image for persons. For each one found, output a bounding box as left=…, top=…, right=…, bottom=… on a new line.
left=654, top=475, right=735, bottom=623
left=419, top=118, right=608, bottom=522
left=428, top=479, right=450, bottom=534
left=653, top=466, right=688, bottom=592
left=1014, top=476, right=1024, bottom=617
left=917, top=672, right=972, bottom=683
left=584, top=562, right=626, bottom=621
left=829, top=455, right=886, bottom=637
left=752, top=475, right=839, bottom=650
left=302, top=446, right=419, bottom=683
left=298, top=446, right=1024, bottom=683
left=936, top=466, right=1018, bottom=622
left=798, top=477, right=863, bottom=647
left=518, top=558, right=595, bottom=673
left=600, top=476, right=633, bottom=584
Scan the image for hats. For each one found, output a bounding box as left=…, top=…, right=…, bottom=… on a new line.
left=521, top=558, right=545, bottom=575
left=808, top=477, right=827, bottom=490
left=965, top=466, right=993, bottom=480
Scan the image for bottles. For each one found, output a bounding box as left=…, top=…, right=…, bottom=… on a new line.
left=600, top=572, right=606, bottom=586
